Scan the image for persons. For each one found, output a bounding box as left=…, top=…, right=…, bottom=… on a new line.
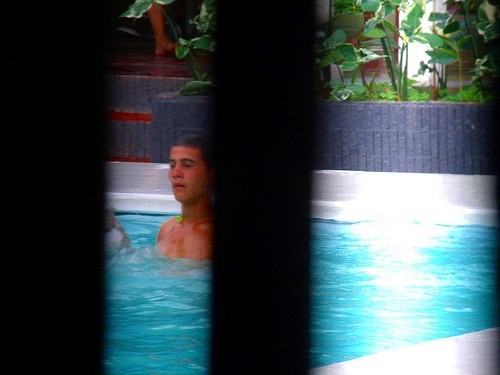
left=156, top=132, right=210, bottom=261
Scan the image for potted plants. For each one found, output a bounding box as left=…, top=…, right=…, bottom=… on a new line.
left=120, top=0, right=498, bottom=175
